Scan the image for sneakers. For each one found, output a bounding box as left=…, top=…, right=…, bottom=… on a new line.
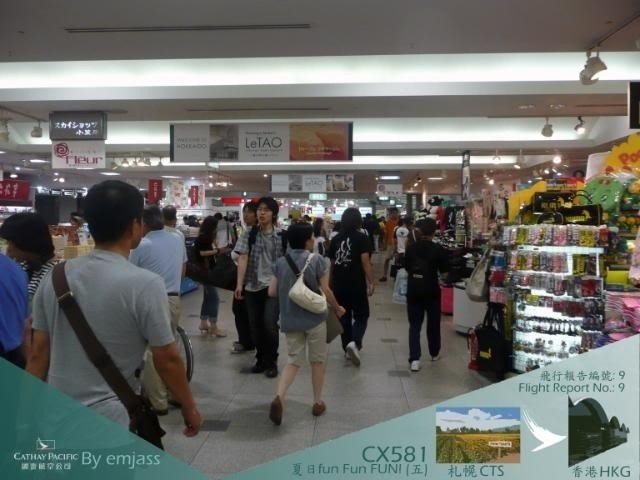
left=379, top=277, right=386, bottom=282
left=230, top=341, right=441, bottom=425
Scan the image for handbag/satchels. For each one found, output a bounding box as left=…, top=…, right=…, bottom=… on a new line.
left=208, top=254, right=238, bottom=291
left=467, top=324, right=504, bottom=372
left=327, top=301, right=343, bottom=345
left=289, top=274, right=327, bottom=314
left=390, top=265, right=404, bottom=278
left=187, top=262, right=208, bottom=284
left=463, top=257, right=491, bottom=302
left=139, top=401, right=164, bottom=453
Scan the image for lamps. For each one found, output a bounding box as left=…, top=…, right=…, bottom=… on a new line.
left=574, top=117, right=586, bottom=136
left=1, top=116, right=232, bottom=187
left=541, top=116, right=553, bottom=138
left=407, top=150, right=561, bottom=192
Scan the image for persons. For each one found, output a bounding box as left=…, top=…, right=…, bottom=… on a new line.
left=15, top=180, right=202, bottom=480
left=0, top=207, right=188, bottom=416
left=233, top=197, right=290, bottom=378
left=405, top=218, right=450, bottom=371
left=267, top=222, right=346, bottom=425
left=330, top=208, right=375, bottom=367
left=214, top=202, right=459, bottom=352
left=194, top=215, right=226, bottom=337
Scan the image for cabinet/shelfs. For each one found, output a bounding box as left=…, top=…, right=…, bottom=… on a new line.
left=511, top=244, right=605, bottom=375
left=1, top=201, right=34, bottom=232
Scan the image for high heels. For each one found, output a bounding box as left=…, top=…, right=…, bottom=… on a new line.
left=200, top=326, right=227, bottom=338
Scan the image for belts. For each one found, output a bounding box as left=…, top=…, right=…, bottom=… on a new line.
left=168, top=292, right=179, bottom=296
